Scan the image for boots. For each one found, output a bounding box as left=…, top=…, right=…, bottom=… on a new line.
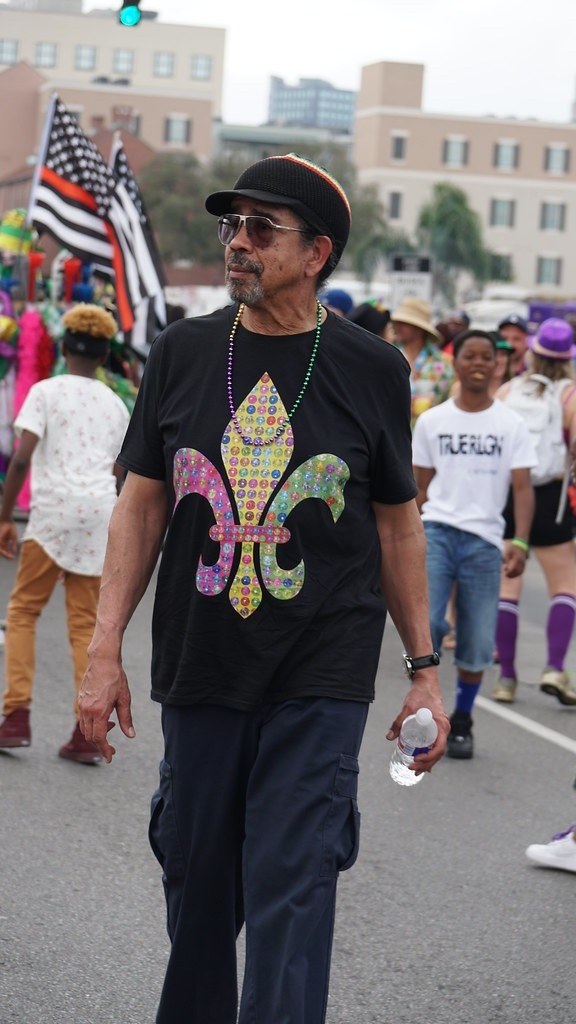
left=0, top=706, right=30, bottom=748
left=58, top=717, right=115, bottom=762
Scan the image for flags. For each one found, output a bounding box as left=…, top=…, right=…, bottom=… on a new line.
left=30, top=95, right=169, bottom=357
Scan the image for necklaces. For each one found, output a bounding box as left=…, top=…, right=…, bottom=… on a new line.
left=228, top=298, right=323, bottom=447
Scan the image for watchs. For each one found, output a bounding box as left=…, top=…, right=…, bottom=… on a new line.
left=402, top=652, right=440, bottom=678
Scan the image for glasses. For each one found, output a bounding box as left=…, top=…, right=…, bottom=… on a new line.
left=217, top=213, right=306, bottom=250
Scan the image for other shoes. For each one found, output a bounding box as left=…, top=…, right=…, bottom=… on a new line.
left=442, top=728, right=472, bottom=760
left=525, top=824, right=576, bottom=872
left=540, top=668, right=576, bottom=708
left=492, top=673, right=515, bottom=702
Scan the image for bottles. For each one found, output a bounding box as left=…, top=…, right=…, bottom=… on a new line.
left=389, top=708, right=438, bottom=787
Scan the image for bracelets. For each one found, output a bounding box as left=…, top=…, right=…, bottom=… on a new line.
left=512, top=537, right=529, bottom=553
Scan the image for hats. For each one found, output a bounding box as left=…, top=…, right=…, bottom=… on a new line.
left=206, top=151, right=350, bottom=267
left=499, top=315, right=525, bottom=333
left=527, top=317, right=576, bottom=358
left=487, top=331, right=514, bottom=355
left=390, top=297, right=443, bottom=343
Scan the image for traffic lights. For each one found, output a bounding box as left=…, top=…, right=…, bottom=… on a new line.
left=117, top=0, right=142, bottom=28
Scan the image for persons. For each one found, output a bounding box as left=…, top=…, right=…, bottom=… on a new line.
left=78, top=151, right=451, bottom=1024
left=490, top=315, right=532, bottom=393
left=412, top=329, right=537, bottom=760
left=0, top=303, right=130, bottom=763
left=493, top=318, right=576, bottom=707
left=324, top=289, right=469, bottom=415
left=526, top=780, right=576, bottom=875
